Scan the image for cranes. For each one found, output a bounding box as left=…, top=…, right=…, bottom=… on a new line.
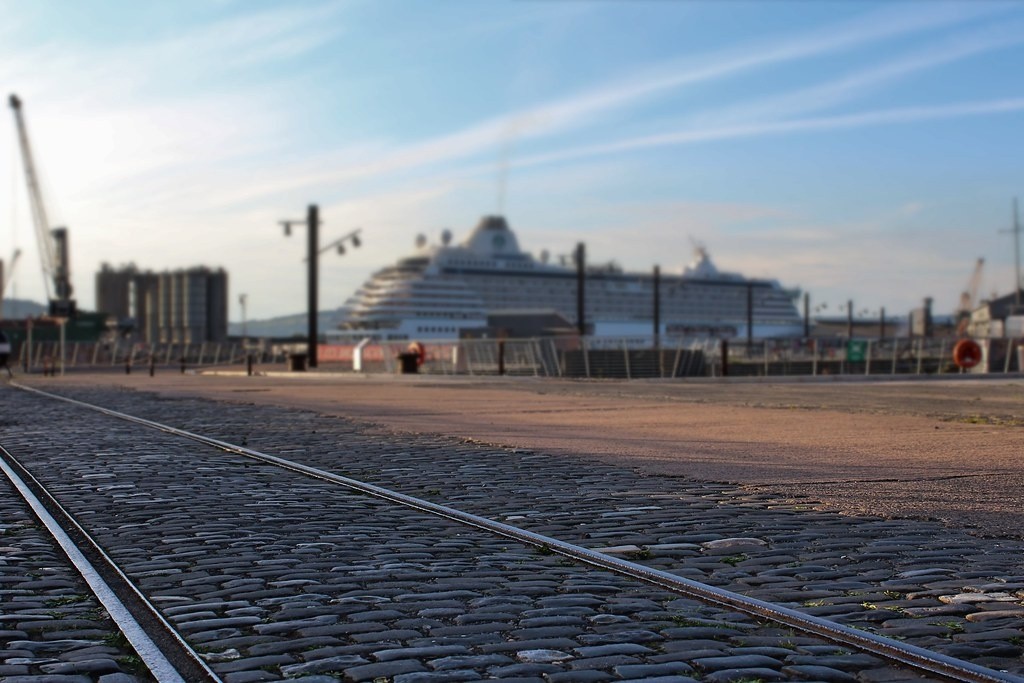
left=7, top=94, right=79, bottom=318
left=950, top=250, right=988, bottom=371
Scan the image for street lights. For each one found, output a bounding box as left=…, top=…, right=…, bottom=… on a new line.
left=280, top=203, right=364, bottom=368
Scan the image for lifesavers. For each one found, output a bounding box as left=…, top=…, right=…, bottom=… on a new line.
left=952, top=339, right=981, bottom=367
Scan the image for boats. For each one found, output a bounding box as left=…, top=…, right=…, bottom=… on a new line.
left=320, top=212, right=814, bottom=372
